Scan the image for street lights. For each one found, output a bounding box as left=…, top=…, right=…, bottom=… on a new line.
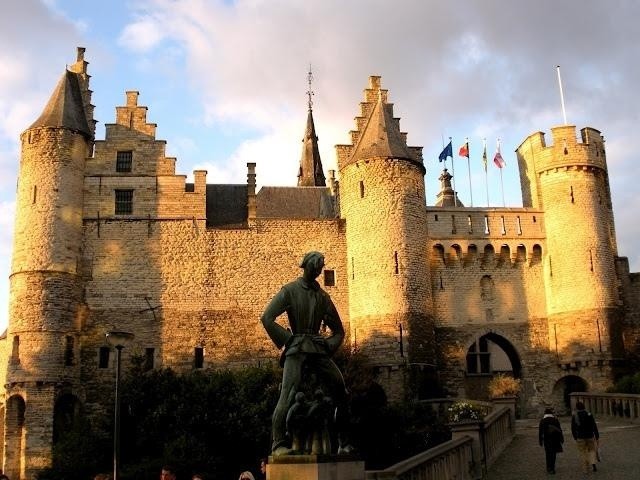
left=104, top=331, right=135, bottom=479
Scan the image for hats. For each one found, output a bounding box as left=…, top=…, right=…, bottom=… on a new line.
left=576, top=402, right=585, bottom=410
left=544, top=408, right=554, bottom=414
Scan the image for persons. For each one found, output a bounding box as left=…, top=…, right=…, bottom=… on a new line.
left=258, top=253, right=364, bottom=461
left=94, top=473, right=115, bottom=480
left=238, top=470, right=254, bottom=480
left=189, top=473, right=203, bottom=480
left=538, top=406, right=564, bottom=475
left=570, top=402, right=601, bottom=475
left=160, top=467, right=174, bottom=480
left=259, top=460, right=266, bottom=476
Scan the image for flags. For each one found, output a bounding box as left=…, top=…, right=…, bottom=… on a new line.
left=494, top=148, right=507, bottom=170
left=438, top=140, right=452, bottom=161
left=458, top=142, right=470, bottom=159
left=482, top=146, right=489, bottom=171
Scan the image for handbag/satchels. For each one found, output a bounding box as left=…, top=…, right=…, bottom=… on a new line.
left=596, top=446, right=600, bottom=462
